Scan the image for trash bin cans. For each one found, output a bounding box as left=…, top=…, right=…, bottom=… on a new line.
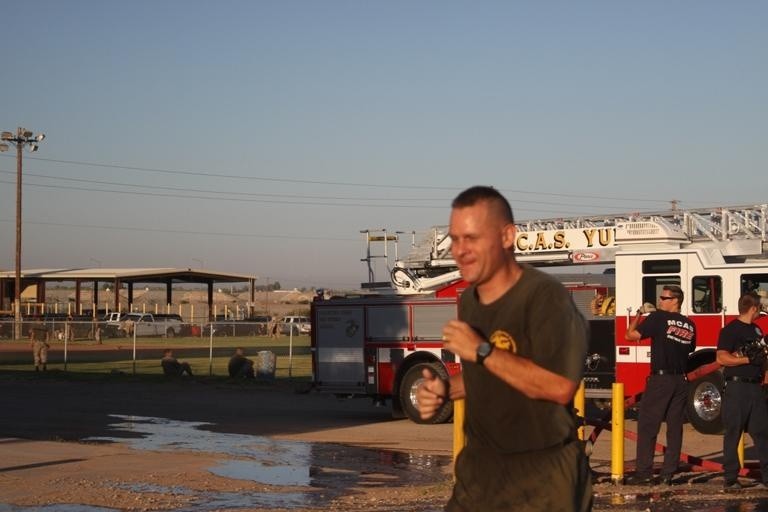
left=256, top=351, right=276, bottom=379
left=191, top=326, right=201, bottom=336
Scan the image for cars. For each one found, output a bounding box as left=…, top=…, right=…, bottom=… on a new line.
left=205, top=316, right=267, bottom=337
left=270, top=315, right=312, bottom=337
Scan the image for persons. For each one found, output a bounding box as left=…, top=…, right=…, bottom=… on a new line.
left=30, top=316, right=48, bottom=371
left=161, top=349, right=193, bottom=376
left=714, top=291, right=768, bottom=489
left=228, top=347, right=253, bottom=378
left=49, top=314, right=102, bottom=345
left=414, top=185, right=597, bottom=510
left=624, top=284, right=697, bottom=484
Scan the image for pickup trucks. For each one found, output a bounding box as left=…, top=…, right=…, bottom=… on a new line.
left=91, top=311, right=156, bottom=338
left=105, top=312, right=182, bottom=339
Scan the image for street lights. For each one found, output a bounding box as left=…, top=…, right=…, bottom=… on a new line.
left=0, top=127, right=44, bottom=340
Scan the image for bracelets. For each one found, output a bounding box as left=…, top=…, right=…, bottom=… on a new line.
left=636, top=309, right=642, bottom=314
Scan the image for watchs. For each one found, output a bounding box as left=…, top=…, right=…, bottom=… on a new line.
left=476, top=339, right=492, bottom=366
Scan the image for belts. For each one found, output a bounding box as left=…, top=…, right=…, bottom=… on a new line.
left=653, top=368, right=681, bottom=375
left=727, top=376, right=761, bottom=383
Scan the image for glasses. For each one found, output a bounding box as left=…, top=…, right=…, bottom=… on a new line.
left=660, top=296, right=674, bottom=300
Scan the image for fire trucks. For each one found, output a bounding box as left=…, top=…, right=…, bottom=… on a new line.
left=309, top=203, right=768, bottom=434
